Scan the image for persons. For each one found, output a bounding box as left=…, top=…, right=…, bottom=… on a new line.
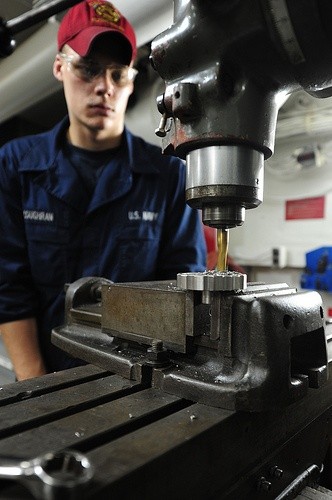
left=2, top=0, right=207, bottom=385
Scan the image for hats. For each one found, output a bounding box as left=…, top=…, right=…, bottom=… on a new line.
left=57, top=0, right=137, bottom=60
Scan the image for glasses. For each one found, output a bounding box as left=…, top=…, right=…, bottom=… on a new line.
left=56, top=53, right=139, bottom=87
